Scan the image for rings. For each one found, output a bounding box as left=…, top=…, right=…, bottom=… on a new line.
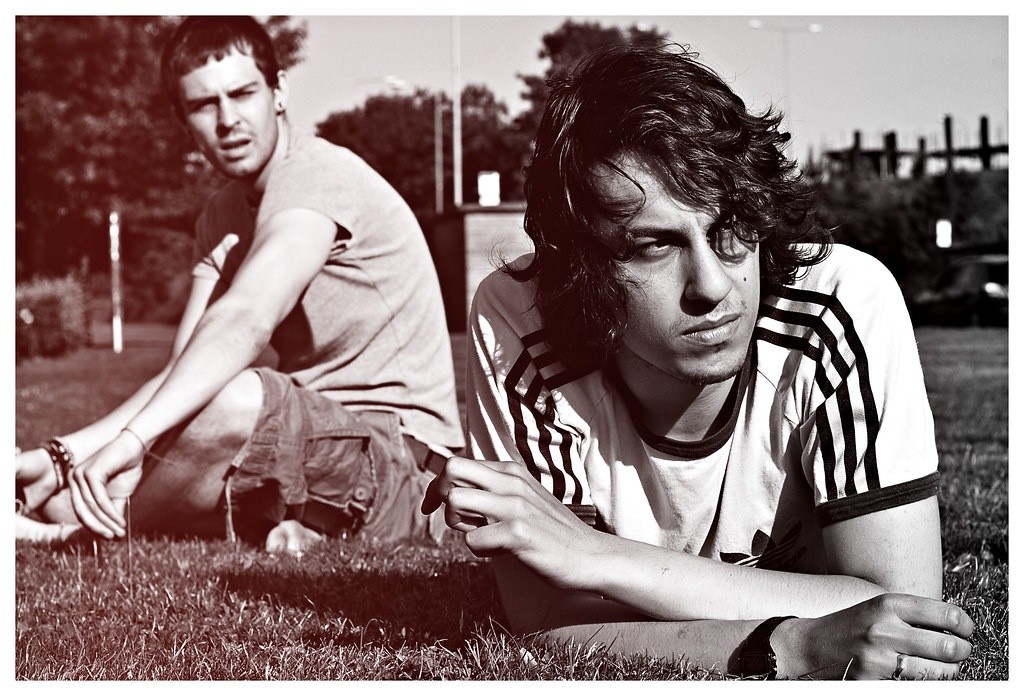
left=892, top=654, right=905, bottom=680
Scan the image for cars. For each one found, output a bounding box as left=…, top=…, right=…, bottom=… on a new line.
left=911, top=256, right=1009, bottom=326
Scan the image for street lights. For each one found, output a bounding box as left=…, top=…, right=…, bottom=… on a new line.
left=747, top=20, right=820, bottom=169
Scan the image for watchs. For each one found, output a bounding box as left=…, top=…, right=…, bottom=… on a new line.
left=738, top=616, right=799, bottom=681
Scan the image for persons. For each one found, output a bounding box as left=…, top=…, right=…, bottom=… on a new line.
left=420, top=42, right=974, bottom=681
left=16, top=15, right=467, bottom=545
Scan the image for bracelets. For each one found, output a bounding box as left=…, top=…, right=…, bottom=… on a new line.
left=121, top=428, right=148, bottom=453
left=38, top=436, right=75, bottom=489
